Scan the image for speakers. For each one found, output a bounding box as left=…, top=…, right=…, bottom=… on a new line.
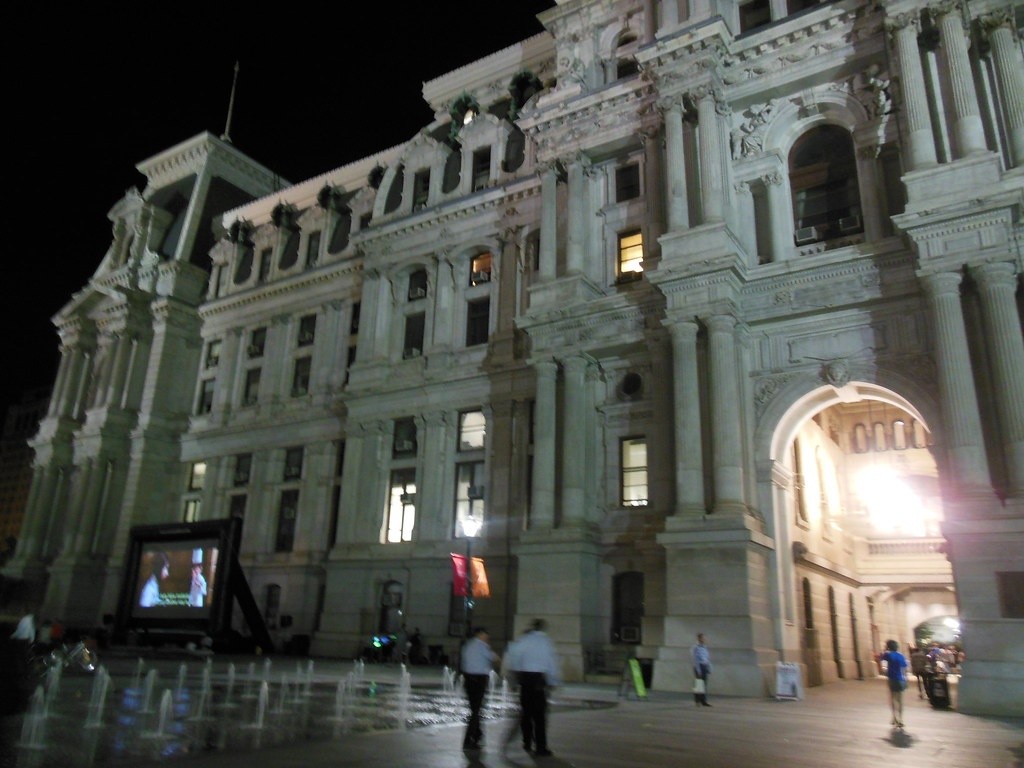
left=104, top=615, right=114, bottom=625
left=281, top=615, right=293, bottom=627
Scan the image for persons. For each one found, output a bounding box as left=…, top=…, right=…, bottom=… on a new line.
left=553, top=57, right=586, bottom=86
left=824, top=362, right=852, bottom=389
left=877, top=639, right=964, bottom=727
left=693, top=633, right=710, bottom=708
left=0, top=574, right=99, bottom=768
left=729, top=102, right=772, bottom=159
left=454, top=617, right=555, bottom=757
left=852, top=63, right=892, bottom=119
left=366, top=622, right=424, bottom=666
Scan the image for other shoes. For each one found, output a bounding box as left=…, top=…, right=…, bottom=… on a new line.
left=702, top=702, right=711, bottom=706
left=898, top=723, right=904, bottom=727
left=535, top=749, right=554, bottom=757
left=920, top=695, right=924, bottom=699
left=522, top=742, right=532, bottom=749
left=890, top=720, right=898, bottom=725
left=464, top=739, right=480, bottom=749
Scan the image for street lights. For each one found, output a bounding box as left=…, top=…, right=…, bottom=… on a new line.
left=454, top=513, right=479, bottom=684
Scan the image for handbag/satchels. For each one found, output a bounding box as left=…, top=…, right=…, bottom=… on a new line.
left=691, top=678, right=705, bottom=694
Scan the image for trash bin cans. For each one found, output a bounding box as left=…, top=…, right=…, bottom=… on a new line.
left=287, top=633, right=311, bottom=659
left=428, top=642, right=444, bottom=665
left=928, top=677, right=952, bottom=708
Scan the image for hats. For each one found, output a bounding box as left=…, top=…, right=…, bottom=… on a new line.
left=932, top=643, right=939, bottom=646
